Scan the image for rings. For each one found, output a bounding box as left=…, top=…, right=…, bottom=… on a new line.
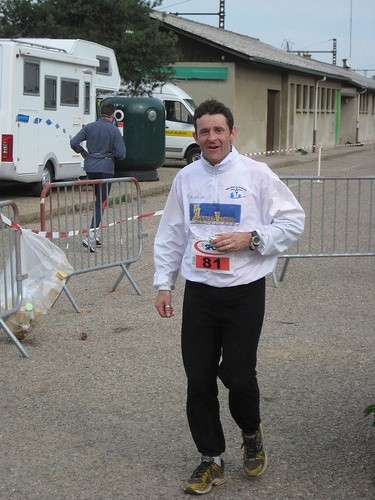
left=228, top=246, right=232, bottom=249
left=166, top=305, right=171, bottom=308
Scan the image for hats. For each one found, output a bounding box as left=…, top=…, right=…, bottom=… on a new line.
left=101, top=103, right=115, bottom=116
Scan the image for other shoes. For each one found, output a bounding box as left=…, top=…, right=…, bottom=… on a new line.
left=83, top=238, right=96, bottom=252
left=95, top=232, right=103, bottom=245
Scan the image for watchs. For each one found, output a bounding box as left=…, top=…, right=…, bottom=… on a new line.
left=251, top=230, right=261, bottom=250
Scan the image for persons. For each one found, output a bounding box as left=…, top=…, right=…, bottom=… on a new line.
left=153, top=99, right=306, bottom=495
left=70, top=103, right=125, bottom=254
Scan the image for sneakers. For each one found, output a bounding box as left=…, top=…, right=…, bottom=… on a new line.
left=239, top=424, right=267, bottom=477
left=183, top=453, right=228, bottom=494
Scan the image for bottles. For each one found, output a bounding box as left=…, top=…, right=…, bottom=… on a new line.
left=0, top=295, right=43, bottom=340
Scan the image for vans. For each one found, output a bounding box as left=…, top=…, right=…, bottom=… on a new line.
left=0, top=35, right=121, bottom=198
left=119, top=77, right=240, bottom=169
